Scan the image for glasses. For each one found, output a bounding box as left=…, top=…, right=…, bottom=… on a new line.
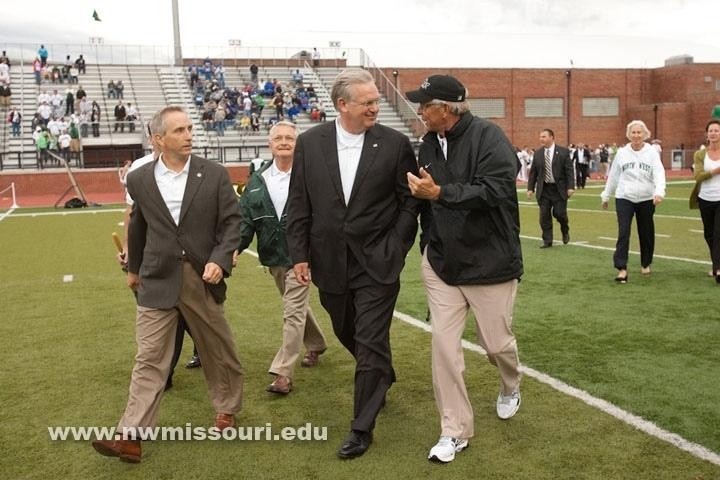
left=352, top=99, right=382, bottom=107
left=270, top=136, right=295, bottom=143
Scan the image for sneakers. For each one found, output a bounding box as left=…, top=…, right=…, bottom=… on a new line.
left=427, top=436, right=469, bottom=463
left=614, top=273, right=629, bottom=284
left=496, top=388, right=522, bottom=419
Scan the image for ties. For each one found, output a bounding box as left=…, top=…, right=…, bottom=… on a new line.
left=545, top=150, right=551, bottom=183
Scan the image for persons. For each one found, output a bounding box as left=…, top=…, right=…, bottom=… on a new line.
left=568, top=143, right=577, bottom=183
left=74, top=55, right=85, bottom=73
left=68, top=114, right=80, bottom=124
left=118, top=160, right=132, bottom=226
left=60, top=67, right=71, bottom=84
left=38, top=44, right=48, bottom=67
left=0, top=58, right=9, bottom=74
left=115, top=80, right=125, bottom=98
left=62, top=55, right=71, bottom=72
left=113, top=100, right=127, bottom=133
left=33, top=127, right=58, bottom=169
left=511, top=144, right=536, bottom=182
left=42, top=64, right=54, bottom=82
left=528, top=129, right=574, bottom=248
left=250, top=154, right=266, bottom=175
left=58, top=130, right=72, bottom=164
left=124, top=103, right=138, bottom=132
left=284, top=69, right=422, bottom=458
left=583, top=142, right=618, bottom=179
left=0, top=71, right=11, bottom=112
left=652, top=140, right=662, bottom=160
left=92, top=108, right=242, bottom=463
left=107, top=80, right=116, bottom=98
left=92, top=100, right=101, bottom=122
left=8, top=107, right=22, bottom=137
left=600, top=120, right=666, bottom=284
left=92, top=109, right=100, bottom=137
left=406, top=74, right=524, bottom=461
left=188, top=56, right=326, bottom=136
left=31, top=90, right=69, bottom=129
left=119, top=110, right=203, bottom=391
left=33, top=56, right=41, bottom=84
left=66, top=85, right=76, bottom=115
left=69, top=122, right=79, bottom=158
left=690, top=121, right=720, bottom=284
left=76, top=86, right=87, bottom=116
left=0, top=51, right=10, bottom=66
left=70, top=64, right=79, bottom=84
left=573, top=142, right=592, bottom=189
left=232, top=121, right=328, bottom=393
left=80, top=97, right=88, bottom=112
left=80, top=110, right=89, bottom=137
left=52, top=64, right=63, bottom=83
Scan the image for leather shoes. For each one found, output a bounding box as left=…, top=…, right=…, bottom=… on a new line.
left=562, top=234, right=570, bottom=245
left=540, top=243, right=552, bottom=249
left=185, top=355, right=201, bottom=369
left=214, top=414, right=235, bottom=432
left=92, top=433, right=141, bottom=464
left=301, top=351, right=319, bottom=367
left=337, top=431, right=373, bottom=459
left=265, top=376, right=294, bottom=394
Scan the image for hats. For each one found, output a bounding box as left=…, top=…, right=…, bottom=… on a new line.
left=405, top=75, right=466, bottom=104
